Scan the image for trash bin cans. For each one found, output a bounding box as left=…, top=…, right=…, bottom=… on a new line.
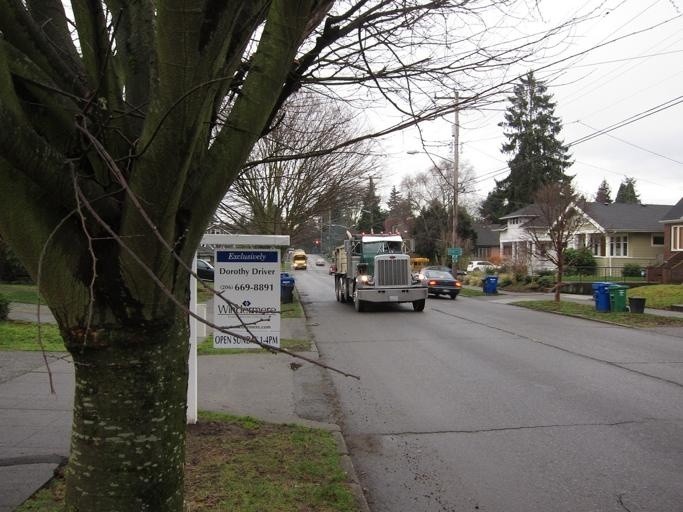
left=281, top=272, right=294, bottom=303
left=591, top=281, right=646, bottom=313
left=481, top=277, right=497, bottom=294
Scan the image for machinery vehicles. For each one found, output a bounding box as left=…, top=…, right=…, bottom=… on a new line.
left=290, top=248, right=308, bottom=270
left=329, top=228, right=428, bottom=312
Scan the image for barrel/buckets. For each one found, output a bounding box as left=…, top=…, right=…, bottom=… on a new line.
left=628, top=294, right=647, bottom=313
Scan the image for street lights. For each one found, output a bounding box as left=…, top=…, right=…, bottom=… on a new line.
left=407, top=149, right=459, bottom=279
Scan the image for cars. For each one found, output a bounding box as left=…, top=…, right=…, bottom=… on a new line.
left=316, top=259, right=325, bottom=266
left=467, top=260, right=501, bottom=272
left=329, top=264, right=336, bottom=275
left=410, top=257, right=466, bottom=299
left=198, top=259, right=214, bottom=279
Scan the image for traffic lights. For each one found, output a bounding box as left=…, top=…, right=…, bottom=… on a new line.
left=313, top=241, right=321, bottom=244
